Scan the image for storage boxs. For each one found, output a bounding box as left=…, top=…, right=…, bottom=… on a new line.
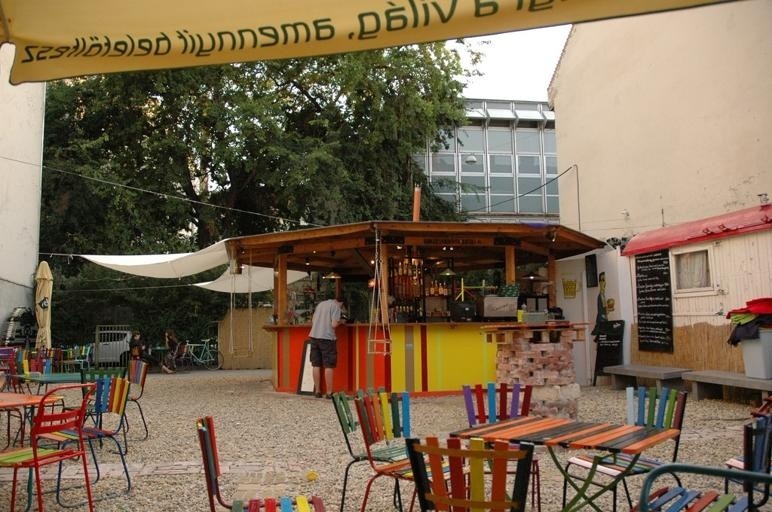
left=740, top=330, right=772, bottom=379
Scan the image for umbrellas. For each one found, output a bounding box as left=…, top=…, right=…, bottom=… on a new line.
left=35, top=261, right=53, bottom=351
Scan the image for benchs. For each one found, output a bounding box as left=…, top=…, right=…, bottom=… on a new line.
left=681, top=369, right=743, bottom=401
left=603, top=364, right=693, bottom=399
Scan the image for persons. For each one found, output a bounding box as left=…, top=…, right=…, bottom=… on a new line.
left=308, top=296, right=347, bottom=400
left=591, top=271, right=618, bottom=336
left=129, top=331, right=188, bottom=374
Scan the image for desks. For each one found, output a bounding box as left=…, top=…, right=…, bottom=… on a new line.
left=0, top=391, right=64, bottom=450
left=449, top=415, right=681, bottom=512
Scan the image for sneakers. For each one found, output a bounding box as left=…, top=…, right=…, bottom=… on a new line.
left=326, top=392, right=334, bottom=399
left=315, top=392, right=324, bottom=399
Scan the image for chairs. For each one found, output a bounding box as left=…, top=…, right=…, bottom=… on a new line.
left=57, top=382, right=128, bottom=462
left=194, top=414, right=329, bottom=511
left=0, top=344, right=95, bottom=394
left=27, top=377, right=133, bottom=509
left=0, top=383, right=97, bottom=512
left=354, top=391, right=471, bottom=512
left=562, top=386, right=689, bottom=512
left=330, top=386, right=428, bottom=512
left=405, top=437, right=534, bottom=512
left=87, top=359, right=149, bottom=438
left=724, top=411, right=772, bottom=508
left=629, top=457, right=772, bottom=512
left=462, top=382, right=541, bottom=512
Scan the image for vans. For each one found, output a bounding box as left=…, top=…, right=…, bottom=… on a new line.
left=85, top=330, right=132, bottom=366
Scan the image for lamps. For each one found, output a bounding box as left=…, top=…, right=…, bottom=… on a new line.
left=324, top=269, right=342, bottom=279
left=438, top=257, right=457, bottom=276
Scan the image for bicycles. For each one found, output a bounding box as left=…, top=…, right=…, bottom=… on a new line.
left=173, top=339, right=225, bottom=371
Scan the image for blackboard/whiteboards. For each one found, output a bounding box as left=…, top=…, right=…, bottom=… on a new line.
left=636, top=249, right=673, bottom=353
left=593, top=320, right=624, bottom=385
